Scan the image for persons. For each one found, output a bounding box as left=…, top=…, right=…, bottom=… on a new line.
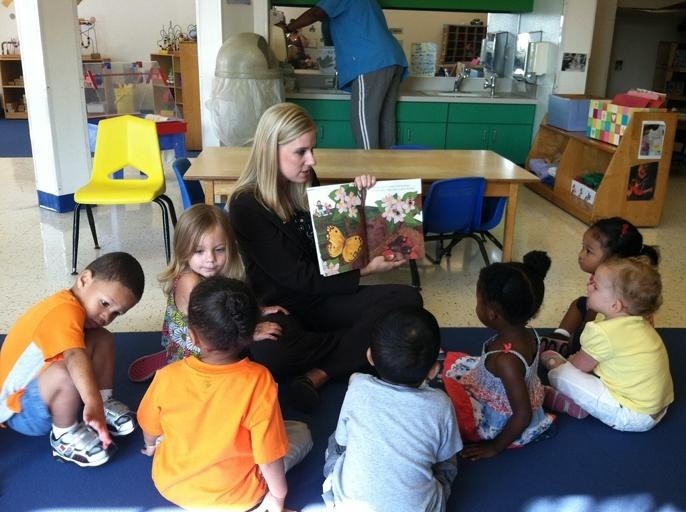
left=225, top=100, right=427, bottom=408
left=543, top=217, right=661, bottom=364
left=137, top=277, right=312, bottom=512
left=0, top=248, right=144, bottom=469
left=126, top=202, right=332, bottom=387
left=536, top=256, right=677, bottom=434
left=271, top=0, right=411, bottom=150
left=439, top=249, right=555, bottom=463
left=630, top=165, right=653, bottom=197
left=319, top=305, right=463, bottom=512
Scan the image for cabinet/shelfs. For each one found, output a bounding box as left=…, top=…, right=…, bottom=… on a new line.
left=444, top=102, right=535, bottom=164
left=176, top=39, right=202, bottom=151
left=394, top=100, right=449, bottom=149
left=525, top=111, right=681, bottom=228
left=148, top=50, right=182, bottom=119
left=0, top=53, right=29, bottom=121
left=284, top=98, right=355, bottom=148
left=80, top=54, right=112, bottom=115
left=438, top=23, right=487, bottom=70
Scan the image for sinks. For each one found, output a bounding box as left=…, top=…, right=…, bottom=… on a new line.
left=424, top=90, right=491, bottom=98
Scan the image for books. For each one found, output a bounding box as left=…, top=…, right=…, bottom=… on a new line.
left=304, top=177, right=427, bottom=277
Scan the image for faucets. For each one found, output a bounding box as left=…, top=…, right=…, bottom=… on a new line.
left=454, top=75, right=466, bottom=93
left=484, top=76, right=496, bottom=96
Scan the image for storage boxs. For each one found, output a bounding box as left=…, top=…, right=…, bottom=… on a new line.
left=570, top=178, right=595, bottom=204
left=588, top=95, right=666, bottom=148
left=547, top=93, right=609, bottom=135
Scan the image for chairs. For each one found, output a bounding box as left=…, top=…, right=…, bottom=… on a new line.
left=169, top=156, right=226, bottom=210
left=440, top=194, right=507, bottom=258
left=71, top=114, right=176, bottom=277
left=410, top=176, right=490, bottom=293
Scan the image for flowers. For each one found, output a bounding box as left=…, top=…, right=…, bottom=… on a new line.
left=327, top=184, right=362, bottom=224
left=373, top=190, right=422, bottom=234
left=321, top=258, right=351, bottom=277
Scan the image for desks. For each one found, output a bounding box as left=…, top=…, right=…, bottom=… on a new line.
left=182, top=147, right=541, bottom=264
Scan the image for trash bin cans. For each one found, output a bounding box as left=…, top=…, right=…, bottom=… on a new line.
left=215, top=33, right=284, bottom=148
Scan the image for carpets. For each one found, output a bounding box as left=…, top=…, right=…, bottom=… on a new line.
left=1, top=328, right=684, bottom=512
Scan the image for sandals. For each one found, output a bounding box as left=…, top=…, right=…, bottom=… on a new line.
left=285, top=376, right=320, bottom=410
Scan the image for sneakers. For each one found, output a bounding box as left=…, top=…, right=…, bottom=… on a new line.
left=49, top=420, right=109, bottom=467
left=539, top=332, right=571, bottom=357
left=99, top=397, right=136, bottom=437
left=539, top=349, right=567, bottom=371
left=127, top=350, right=169, bottom=383
left=542, top=385, right=589, bottom=420
